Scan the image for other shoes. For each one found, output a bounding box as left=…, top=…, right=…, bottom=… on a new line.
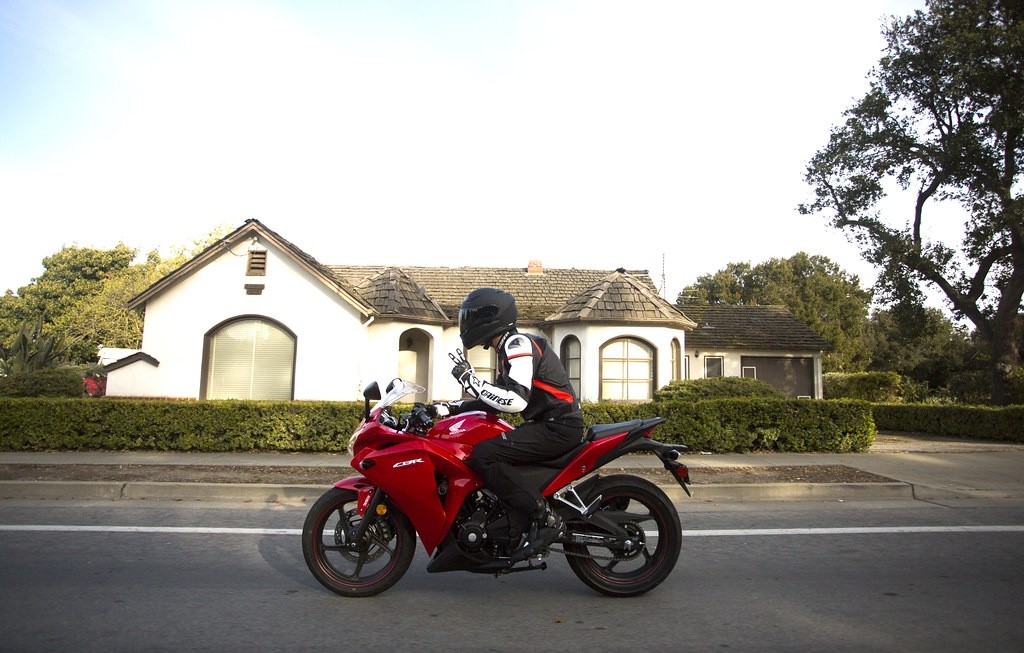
left=528, top=506, right=567, bottom=554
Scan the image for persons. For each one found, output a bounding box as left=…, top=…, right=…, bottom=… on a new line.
left=447, top=288, right=584, bottom=564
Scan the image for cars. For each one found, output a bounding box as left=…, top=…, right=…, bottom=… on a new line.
left=81, top=370, right=107, bottom=397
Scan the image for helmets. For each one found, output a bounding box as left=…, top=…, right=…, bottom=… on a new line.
left=458, top=287, right=517, bottom=349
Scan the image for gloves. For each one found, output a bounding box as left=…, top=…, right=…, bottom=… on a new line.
left=429, top=402, right=451, bottom=417
left=448, top=349, right=474, bottom=384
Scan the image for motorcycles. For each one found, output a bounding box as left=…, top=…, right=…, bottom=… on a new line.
left=300, top=376, right=690, bottom=599
